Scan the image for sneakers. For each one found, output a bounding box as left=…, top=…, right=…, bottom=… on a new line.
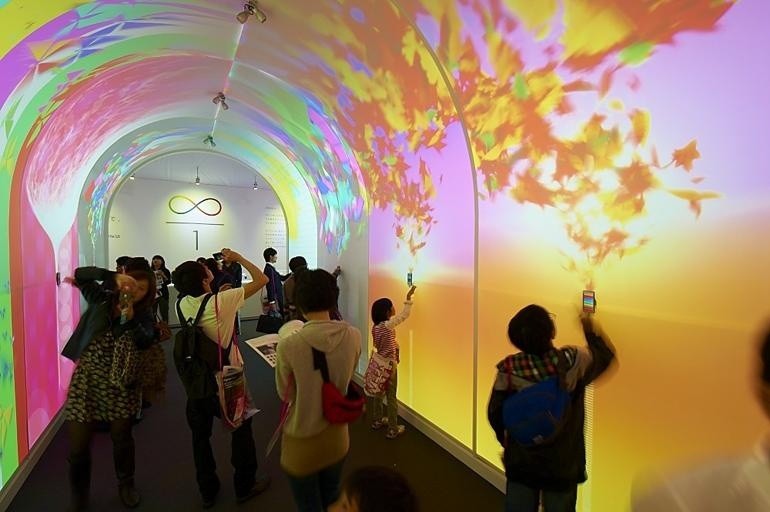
left=237, top=476, right=271, bottom=504
left=372, top=416, right=388, bottom=429
left=386, top=424, right=405, bottom=438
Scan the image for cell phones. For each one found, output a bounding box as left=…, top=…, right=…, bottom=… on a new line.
left=212, top=252, right=225, bottom=261
left=582, top=290, right=595, bottom=314
left=407, top=272, right=412, bottom=286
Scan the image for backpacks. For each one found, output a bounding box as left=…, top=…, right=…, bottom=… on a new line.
left=174, top=294, right=232, bottom=399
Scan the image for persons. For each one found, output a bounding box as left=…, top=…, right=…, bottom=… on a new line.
left=171, top=247, right=270, bottom=511
left=276, top=269, right=361, bottom=510
left=61, top=255, right=171, bottom=511
left=371, top=285, right=416, bottom=439
left=487, top=304, right=615, bottom=512
left=326, top=465, right=419, bottom=511
left=263, top=247, right=343, bottom=321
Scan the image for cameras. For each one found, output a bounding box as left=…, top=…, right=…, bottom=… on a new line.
left=119, top=286, right=133, bottom=308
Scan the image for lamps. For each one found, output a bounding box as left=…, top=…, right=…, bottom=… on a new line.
left=195, top=166, right=201, bottom=186
left=252, top=176, right=259, bottom=190
left=203, top=1, right=267, bottom=149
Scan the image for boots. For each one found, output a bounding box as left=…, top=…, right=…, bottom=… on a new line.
left=113, top=438, right=140, bottom=507
left=70, top=449, right=91, bottom=512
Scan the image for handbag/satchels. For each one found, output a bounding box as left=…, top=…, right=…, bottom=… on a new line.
left=110, top=331, right=138, bottom=390
left=155, top=321, right=171, bottom=341
left=503, top=380, right=564, bottom=446
left=259, top=298, right=280, bottom=314
left=364, top=350, right=392, bottom=397
left=216, top=344, right=261, bottom=429
left=323, top=380, right=368, bottom=422
left=256, top=313, right=284, bottom=334
